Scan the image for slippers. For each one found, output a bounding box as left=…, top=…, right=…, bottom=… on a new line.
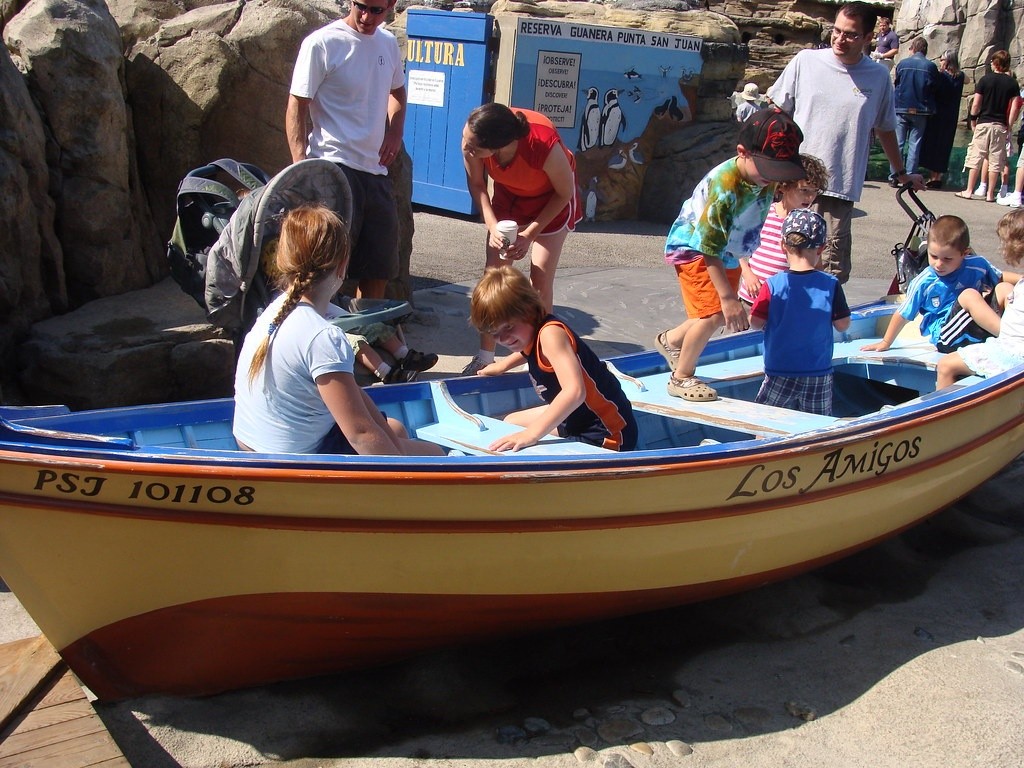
left=955, top=189, right=973, bottom=199
left=986, top=191, right=996, bottom=201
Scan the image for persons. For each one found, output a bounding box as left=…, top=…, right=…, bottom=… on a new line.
left=462, top=103, right=584, bottom=375
left=233, top=207, right=447, bottom=456
left=747, top=208, right=851, bottom=417
left=888, top=37, right=970, bottom=191
left=469, top=266, right=638, bottom=452
left=285, top=0, right=406, bottom=298
left=956, top=51, right=1024, bottom=208
left=766, top=1, right=925, bottom=286
left=720, top=152, right=828, bottom=335
left=735, top=83, right=775, bottom=122
left=870, top=17, right=899, bottom=70
left=261, top=241, right=439, bottom=385
left=860, top=215, right=1024, bottom=351
left=655, top=108, right=809, bottom=402
left=936, top=207, right=1024, bottom=391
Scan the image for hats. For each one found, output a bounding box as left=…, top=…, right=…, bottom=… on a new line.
left=739, top=83, right=761, bottom=101
left=782, top=208, right=827, bottom=249
left=739, top=107, right=808, bottom=181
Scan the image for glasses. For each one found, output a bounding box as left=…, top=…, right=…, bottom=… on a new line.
left=939, top=58, right=948, bottom=62
left=828, top=25, right=869, bottom=43
left=353, top=1, right=387, bottom=14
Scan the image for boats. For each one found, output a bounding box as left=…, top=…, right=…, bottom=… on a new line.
left=0, top=293, right=1024, bottom=705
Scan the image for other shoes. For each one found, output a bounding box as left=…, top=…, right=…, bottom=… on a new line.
left=997, top=192, right=1008, bottom=202
left=383, top=366, right=417, bottom=384
left=924, top=179, right=943, bottom=190
left=1007, top=191, right=1022, bottom=208
left=971, top=191, right=986, bottom=200
left=393, top=348, right=439, bottom=373
left=889, top=179, right=899, bottom=187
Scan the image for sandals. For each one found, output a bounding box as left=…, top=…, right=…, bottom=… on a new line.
left=654, top=329, right=696, bottom=377
left=667, top=372, right=718, bottom=402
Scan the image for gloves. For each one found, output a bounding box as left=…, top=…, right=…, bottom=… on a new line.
left=873, top=53, right=884, bottom=60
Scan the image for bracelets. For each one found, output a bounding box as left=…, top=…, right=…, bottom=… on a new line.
left=971, top=115, right=977, bottom=120
left=895, top=170, right=906, bottom=177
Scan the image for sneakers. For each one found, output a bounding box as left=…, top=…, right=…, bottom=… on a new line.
left=462, top=354, right=497, bottom=376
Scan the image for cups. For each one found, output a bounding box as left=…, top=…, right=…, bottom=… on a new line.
left=496, top=219, right=520, bottom=260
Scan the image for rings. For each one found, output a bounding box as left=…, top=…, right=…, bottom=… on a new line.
left=515, top=249, right=518, bottom=253
left=390, top=153, right=393, bottom=156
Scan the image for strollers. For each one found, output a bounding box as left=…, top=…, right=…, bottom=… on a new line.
left=887, top=181, right=978, bottom=296
left=166, top=158, right=418, bottom=382
